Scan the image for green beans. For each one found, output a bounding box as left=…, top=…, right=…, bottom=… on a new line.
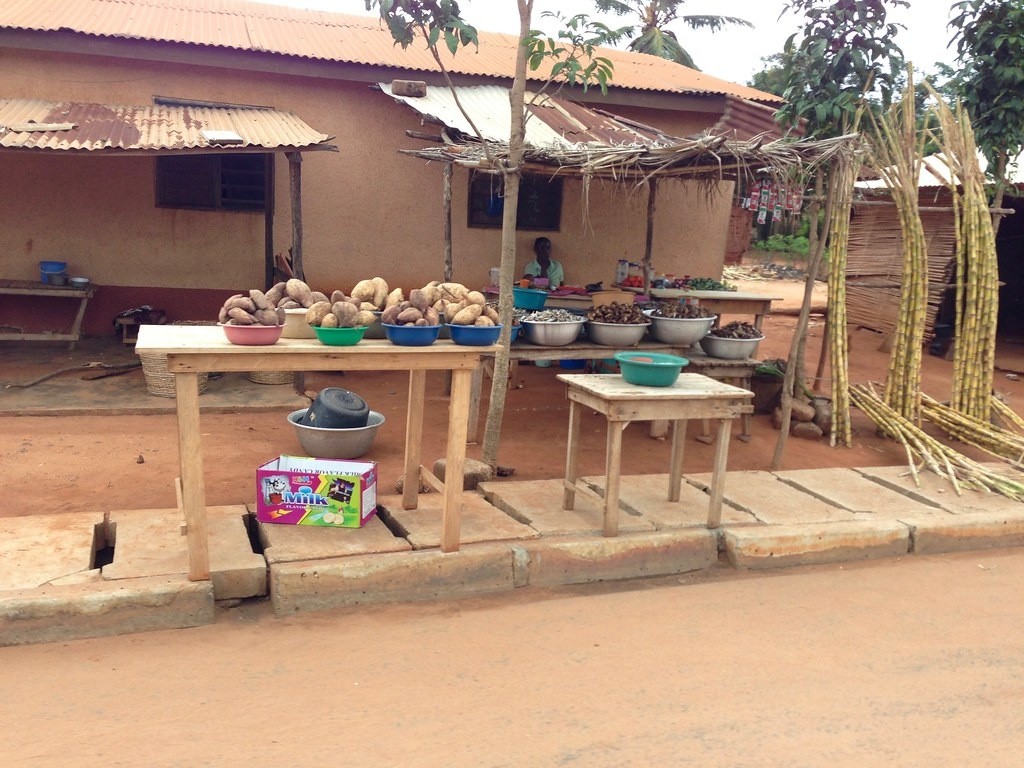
left=687, top=278, right=736, bottom=290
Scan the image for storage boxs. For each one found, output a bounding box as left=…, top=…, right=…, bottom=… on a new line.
left=251, top=454, right=383, bottom=529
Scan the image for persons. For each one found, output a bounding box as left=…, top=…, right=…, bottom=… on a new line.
left=524, top=237, right=565, bottom=291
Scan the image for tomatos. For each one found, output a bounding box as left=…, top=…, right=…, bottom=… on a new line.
left=622, top=275, right=652, bottom=288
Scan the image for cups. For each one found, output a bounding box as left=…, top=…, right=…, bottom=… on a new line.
left=520, top=279, right=530, bottom=288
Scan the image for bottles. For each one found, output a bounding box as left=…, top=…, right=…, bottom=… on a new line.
left=616, top=259, right=638, bottom=284
left=650, top=268, right=656, bottom=277
left=655, top=272, right=691, bottom=290
left=638, top=266, right=644, bottom=277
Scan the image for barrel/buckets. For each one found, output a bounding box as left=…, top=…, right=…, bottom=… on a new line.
left=536, top=360, right=550, bottom=367
left=490, top=268, right=500, bottom=287
left=593, top=358, right=615, bottom=373
left=559, top=359, right=586, bottom=370
left=295, top=387, right=370, bottom=428
left=40, top=260, right=68, bottom=286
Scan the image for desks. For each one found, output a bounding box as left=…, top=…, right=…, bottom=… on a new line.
left=684, top=342, right=764, bottom=437
left=558, top=373, right=756, bottom=540
left=467, top=335, right=690, bottom=444
left=612, top=283, right=785, bottom=360
left=132, top=324, right=506, bottom=581
left=485, top=289, right=593, bottom=389
left=0, top=280, right=98, bottom=347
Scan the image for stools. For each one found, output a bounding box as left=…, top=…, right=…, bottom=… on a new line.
left=116, top=318, right=138, bottom=346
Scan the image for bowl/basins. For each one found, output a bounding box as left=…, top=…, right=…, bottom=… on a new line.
left=217, top=321, right=287, bottom=345
left=513, top=286, right=550, bottom=310
left=615, top=351, right=689, bottom=386
left=381, top=322, right=443, bottom=346
left=287, top=408, right=385, bottom=458
left=698, top=336, right=765, bottom=359
left=520, top=316, right=588, bottom=346
left=436, top=313, right=451, bottom=338
left=362, top=312, right=387, bottom=339
left=274, top=308, right=317, bottom=338
left=591, top=291, right=636, bottom=308
left=70, top=277, right=89, bottom=287
left=642, top=309, right=718, bottom=344
left=311, top=325, right=368, bottom=345
left=583, top=322, right=652, bottom=346
left=534, top=278, right=549, bottom=287
left=445, top=322, right=522, bottom=346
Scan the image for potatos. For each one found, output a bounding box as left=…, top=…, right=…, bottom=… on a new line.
left=219, top=277, right=500, bottom=329
